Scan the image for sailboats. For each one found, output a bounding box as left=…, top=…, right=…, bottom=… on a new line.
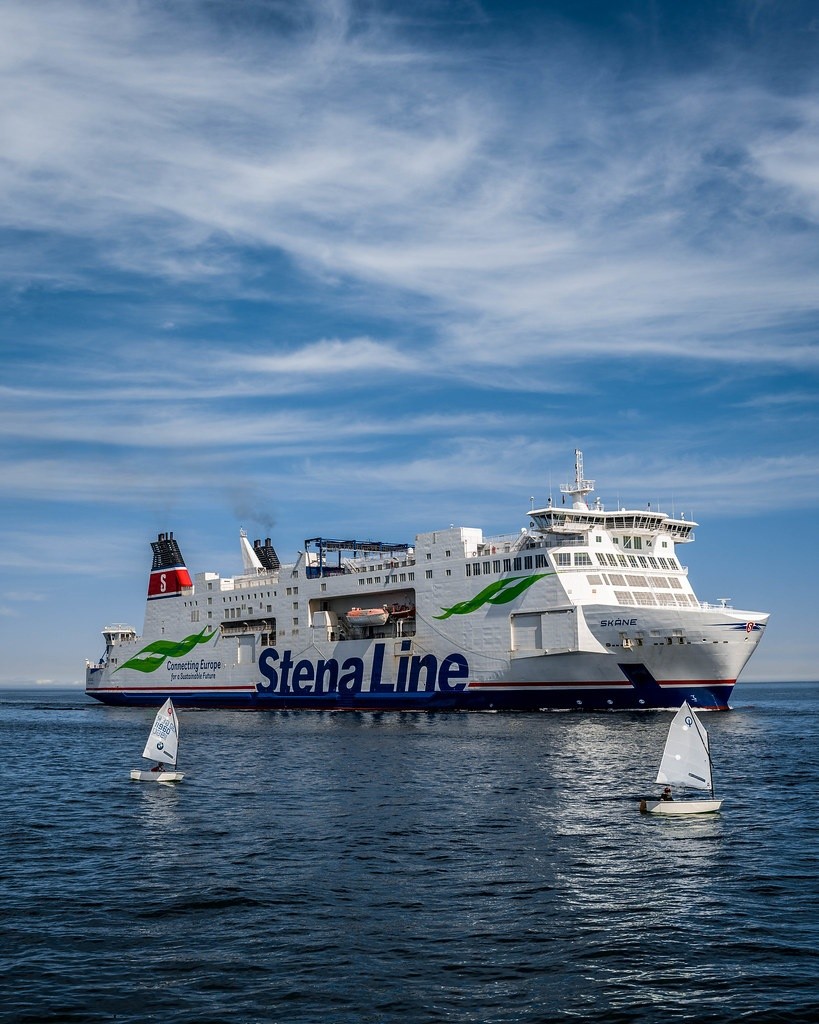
left=639, top=699, right=725, bottom=814
left=131, top=696, right=186, bottom=783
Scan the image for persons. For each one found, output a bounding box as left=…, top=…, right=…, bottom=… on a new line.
left=660, top=788, right=675, bottom=801
left=158, top=762, right=166, bottom=772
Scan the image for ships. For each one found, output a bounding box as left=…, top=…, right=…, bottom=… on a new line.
left=83, top=447, right=771, bottom=714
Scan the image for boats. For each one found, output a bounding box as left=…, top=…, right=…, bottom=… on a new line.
left=389, top=601, right=416, bottom=619
left=345, top=604, right=389, bottom=626
